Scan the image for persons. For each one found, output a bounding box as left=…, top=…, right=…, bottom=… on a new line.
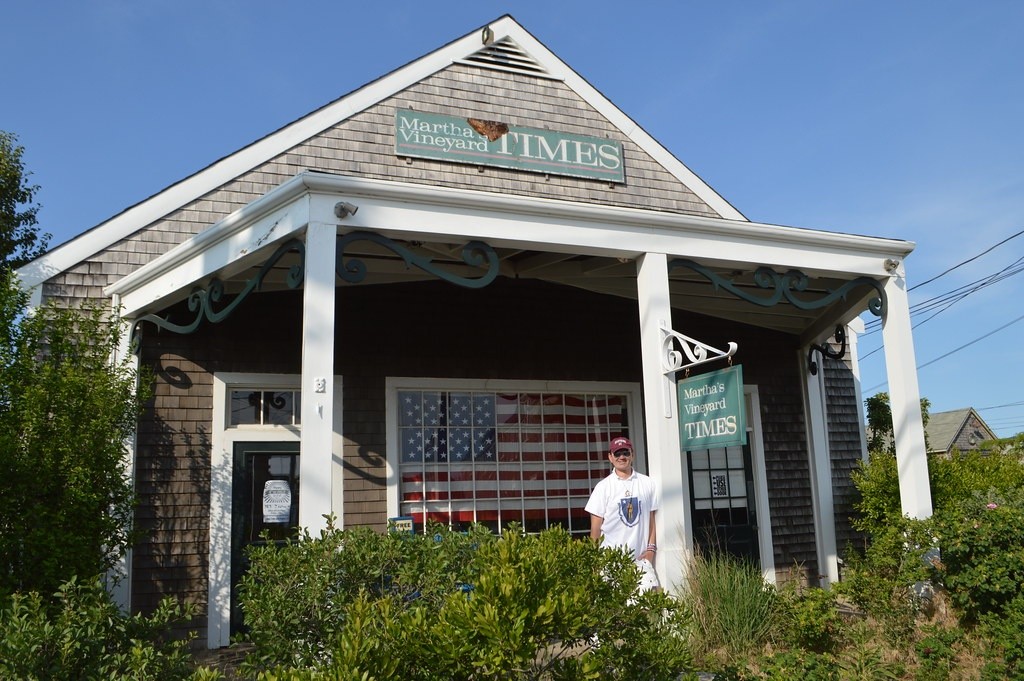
left=584, top=437, right=667, bottom=656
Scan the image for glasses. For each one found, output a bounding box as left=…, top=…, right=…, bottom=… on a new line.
left=614, top=451, right=630, bottom=457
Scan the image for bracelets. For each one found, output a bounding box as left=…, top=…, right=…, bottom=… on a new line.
left=647, top=544, right=655, bottom=552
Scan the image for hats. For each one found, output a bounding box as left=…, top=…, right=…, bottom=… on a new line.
left=609, top=438, right=632, bottom=453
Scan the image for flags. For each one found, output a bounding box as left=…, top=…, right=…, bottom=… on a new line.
left=397, top=390, right=623, bottom=523
